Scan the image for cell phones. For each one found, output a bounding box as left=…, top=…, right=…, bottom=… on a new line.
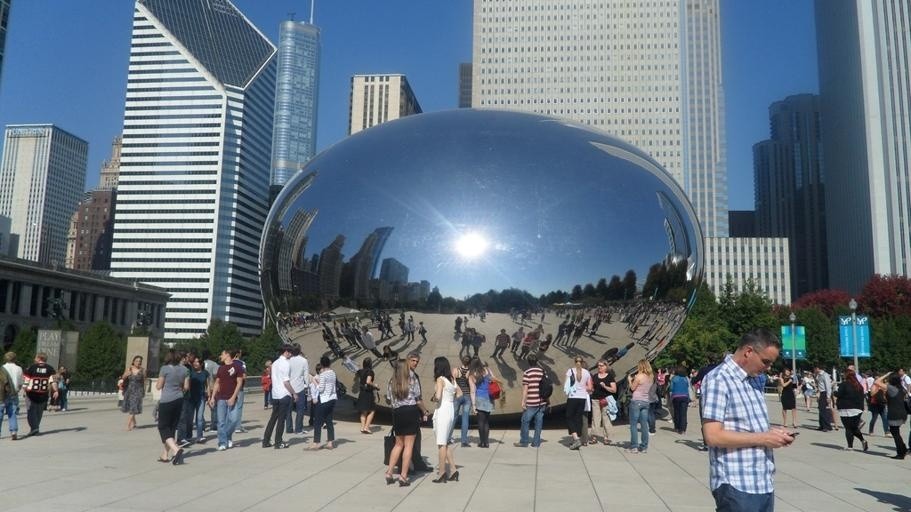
left=789, top=433, right=799, bottom=437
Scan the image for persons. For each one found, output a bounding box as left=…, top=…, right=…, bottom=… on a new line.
left=699, top=326, right=796, bottom=512
left=116, top=342, right=911, bottom=488
left=1, top=350, right=71, bottom=440
left=279, top=300, right=689, bottom=374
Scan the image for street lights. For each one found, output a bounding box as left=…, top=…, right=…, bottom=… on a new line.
left=849, top=298, right=861, bottom=376
left=790, top=311, right=798, bottom=376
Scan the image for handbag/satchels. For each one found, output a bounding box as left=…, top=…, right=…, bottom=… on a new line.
left=539, top=376, right=552, bottom=400
left=904, top=396, right=911, bottom=414
left=385, top=436, right=396, bottom=465
left=336, top=381, right=346, bottom=400
left=488, top=381, right=501, bottom=399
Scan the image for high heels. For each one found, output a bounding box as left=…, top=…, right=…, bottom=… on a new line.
left=385, top=466, right=459, bottom=486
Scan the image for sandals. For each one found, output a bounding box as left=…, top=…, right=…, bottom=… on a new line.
left=569, top=437, right=610, bottom=449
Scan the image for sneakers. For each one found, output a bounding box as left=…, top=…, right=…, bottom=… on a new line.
left=886, top=433, right=892, bottom=437
left=513, top=442, right=538, bottom=446
left=859, top=419, right=865, bottom=430
left=624, top=448, right=646, bottom=453
left=460, top=443, right=489, bottom=448
left=862, top=440, right=867, bottom=451
left=12, top=430, right=17, bottom=439
left=262, top=424, right=333, bottom=450
left=159, top=430, right=247, bottom=464
left=28, top=429, right=38, bottom=436
